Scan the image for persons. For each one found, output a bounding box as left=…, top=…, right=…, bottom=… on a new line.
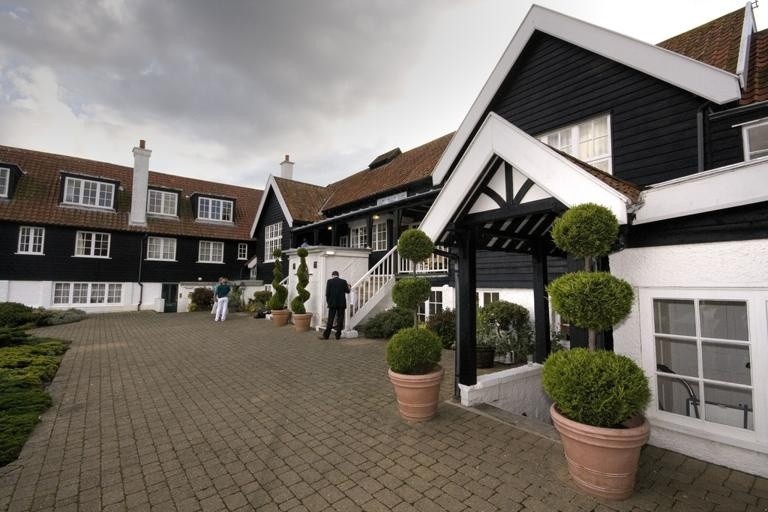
left=214, top=279, right=232, bottom=322
left=318, top=271, right=352, bottom=340
left=210, top=276, right=224, bottom=316
left=332, top=284, right=358, bottom=330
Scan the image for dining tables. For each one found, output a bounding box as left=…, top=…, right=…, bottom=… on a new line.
left=540, top=202, right=650, bottom=501
left=476, top=304, right=496, bottom=369
left=291, top=248, right=313, bottom=331
left=386, top=229, right=444, bottom=423
left=270, top=249, right=290, bottom=328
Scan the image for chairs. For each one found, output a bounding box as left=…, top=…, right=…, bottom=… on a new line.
left=336, top=335, right=341, bottom=340
left=319, top=336, right=330, bottom=340
